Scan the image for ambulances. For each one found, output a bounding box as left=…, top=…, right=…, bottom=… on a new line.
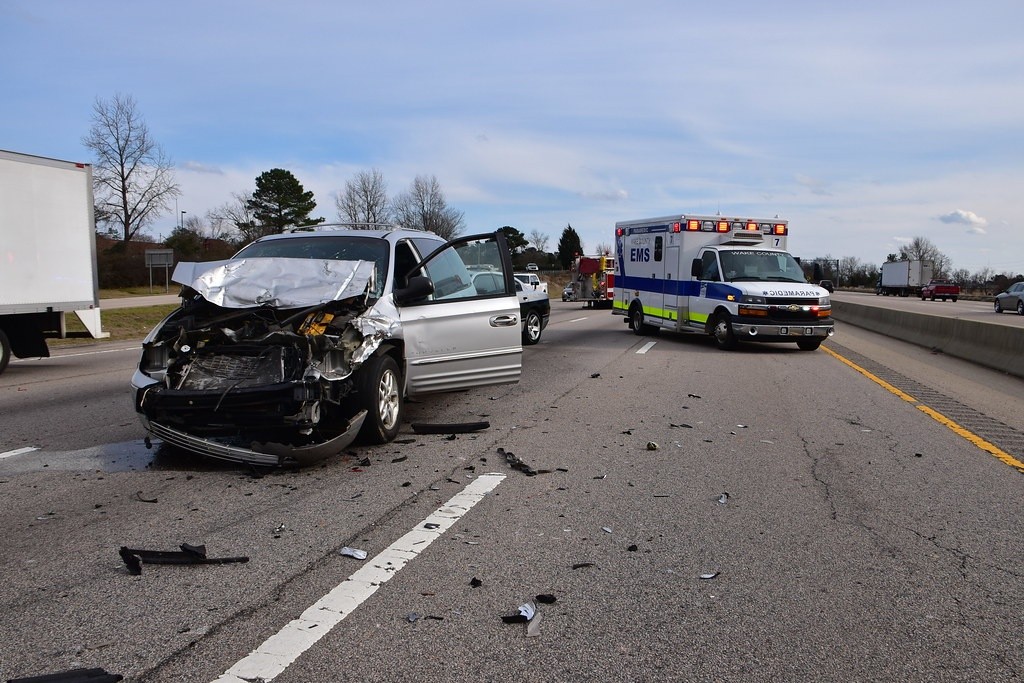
left=612, top=211, right=835, bottom=352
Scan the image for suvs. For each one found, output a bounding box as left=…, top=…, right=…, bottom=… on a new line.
left=130, top=222, right=527, bottom=472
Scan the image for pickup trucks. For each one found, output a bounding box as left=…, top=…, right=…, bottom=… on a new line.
left=513, top=274, right=549, bottom=295
left=921, top=278, right=960, bottom=303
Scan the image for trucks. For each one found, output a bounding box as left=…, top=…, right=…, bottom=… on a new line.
left=875, top=258, right=935, bottom=297
left=0, top=150, right=99, bottom=372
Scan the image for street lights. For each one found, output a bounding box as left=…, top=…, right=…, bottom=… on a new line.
left=181, top=210, right=188, bottom=228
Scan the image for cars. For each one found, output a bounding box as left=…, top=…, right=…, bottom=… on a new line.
left=525, top=263, right=539, bottom=271
left=819, top=280, right=834, bottom=293
left=562, top=282, right=575, bottom=302
left=469, top=271, right=551, bottom=345
left=994, top=281, right=1024, bottom=316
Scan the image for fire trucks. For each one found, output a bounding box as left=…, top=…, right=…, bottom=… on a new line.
left=573, top=251, right=615, bottom=309
